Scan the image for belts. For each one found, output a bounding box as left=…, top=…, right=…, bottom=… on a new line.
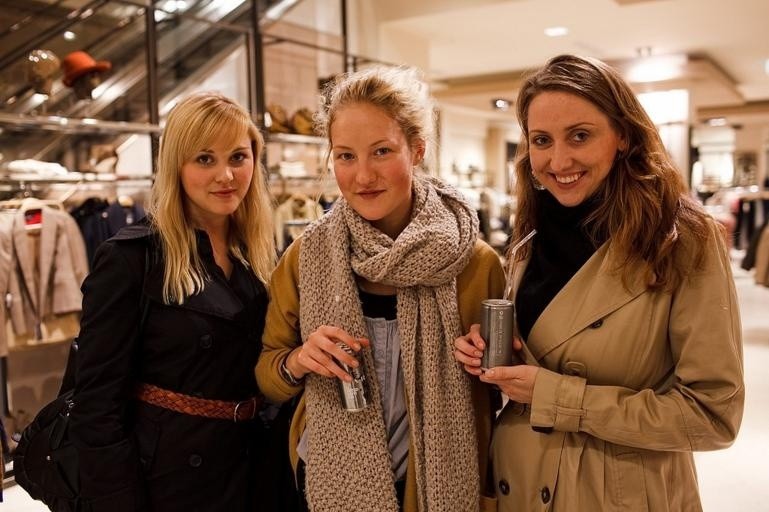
left=136, top=383, right=265, bottom=424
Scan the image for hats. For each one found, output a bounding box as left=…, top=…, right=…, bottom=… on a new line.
left=62, top=50, right=112, bottom=87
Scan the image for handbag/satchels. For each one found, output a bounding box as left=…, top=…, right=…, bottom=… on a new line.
left=13, top=224, right=152, bottom=512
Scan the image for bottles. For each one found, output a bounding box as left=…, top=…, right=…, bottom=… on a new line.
left=331, top=341, right=371, bottom=413
left=480, top=298, right=514, bottom=373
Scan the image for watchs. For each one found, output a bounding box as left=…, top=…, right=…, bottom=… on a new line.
left=280, top=349, right=303, bottom=386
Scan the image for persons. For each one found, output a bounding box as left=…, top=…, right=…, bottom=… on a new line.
left=72, top=88, right=309, bottom=512
left=454, top=53, right=746, bottom=512
left=252, top=68, right=507, bottom=512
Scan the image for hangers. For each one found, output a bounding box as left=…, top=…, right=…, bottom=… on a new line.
left=1, top=197, right=62, bottom=232
